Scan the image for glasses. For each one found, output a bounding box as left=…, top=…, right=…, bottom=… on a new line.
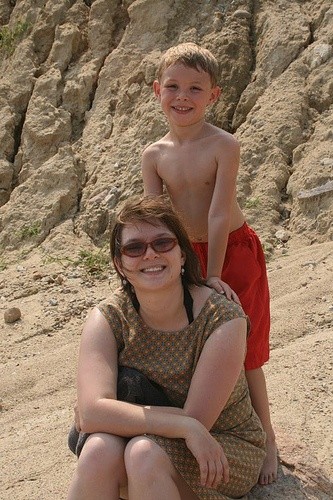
left=120, top=237, right=180, bottom=259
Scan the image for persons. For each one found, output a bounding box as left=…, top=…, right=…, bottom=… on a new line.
left=66, top=199, right=266, bottom=500
left=141, top=42, right=278, bottom=486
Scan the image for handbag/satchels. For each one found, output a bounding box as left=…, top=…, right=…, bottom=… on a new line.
left=67, top=367, right=168, bottom=458
